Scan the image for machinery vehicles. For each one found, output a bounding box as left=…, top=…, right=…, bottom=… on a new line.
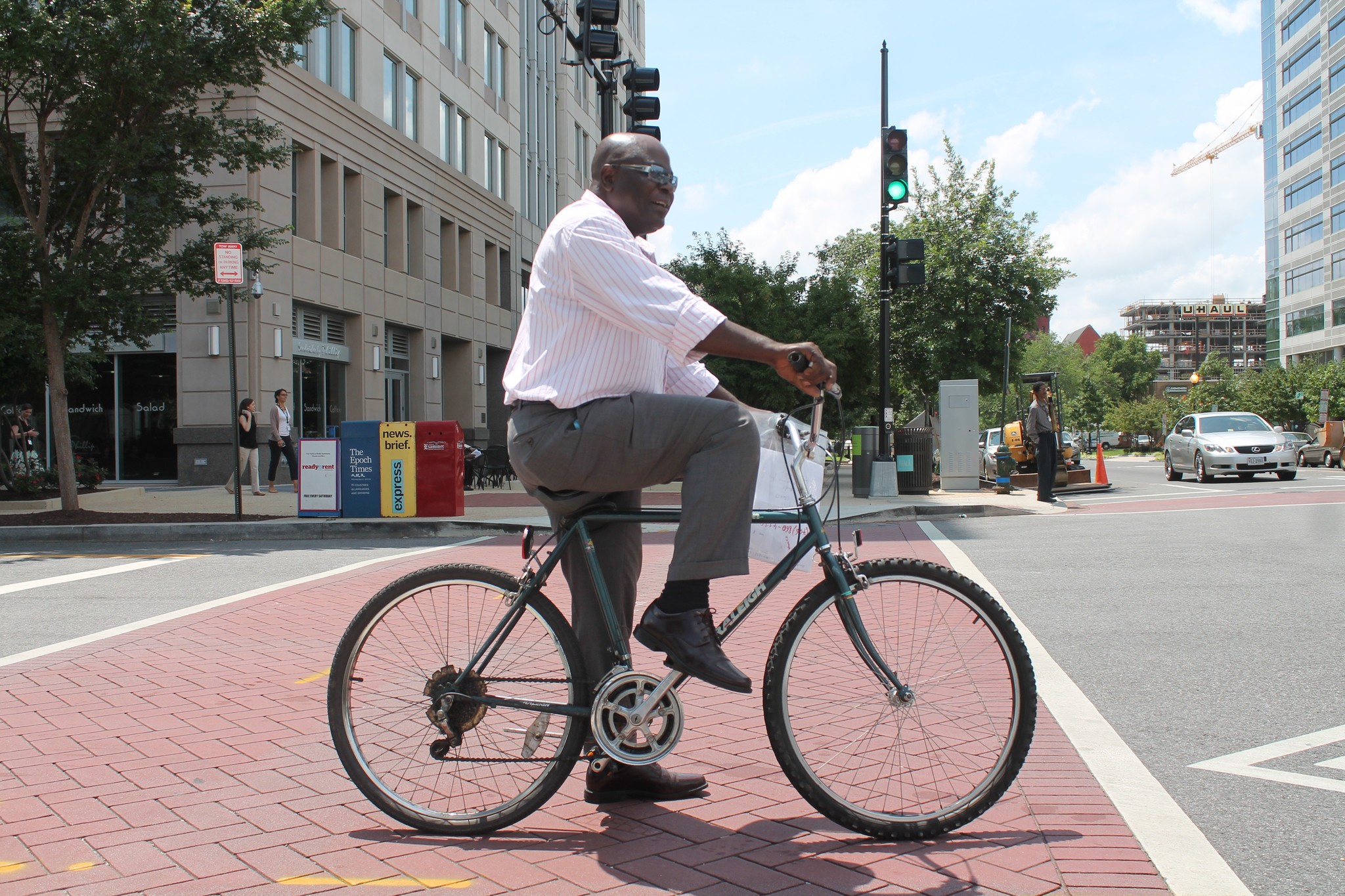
left=1313, top=420, right=1345, bottom=471
left=1004, top=370, right=1116, bottom=495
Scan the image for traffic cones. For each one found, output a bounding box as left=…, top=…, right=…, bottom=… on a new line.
left=1094, top=444, right=1112, bottom=488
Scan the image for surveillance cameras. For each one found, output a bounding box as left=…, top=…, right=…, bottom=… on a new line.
left=250, top=281, right=264, bottom=300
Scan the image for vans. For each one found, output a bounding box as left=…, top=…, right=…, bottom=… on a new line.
left=1136, top=435, right=1152, bottom=448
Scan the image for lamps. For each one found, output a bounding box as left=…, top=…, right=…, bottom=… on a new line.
left=432, top=357, right=439, bottom=378
left=373, top=346, right=380, bottom=370
left=274, top=328, right=283, bottom=357
left=479, top=365, right=484, bottom=384
left=207, top=325, right=219, bottom=356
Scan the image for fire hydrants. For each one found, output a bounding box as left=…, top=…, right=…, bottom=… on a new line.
left=992, top=441, right=1019, bottom=494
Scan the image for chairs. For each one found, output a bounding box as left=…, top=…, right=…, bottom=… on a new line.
left=465, top=445, right=517, bottom=490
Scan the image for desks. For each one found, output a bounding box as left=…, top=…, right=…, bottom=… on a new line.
left=477, top=449, right=499, bottom=486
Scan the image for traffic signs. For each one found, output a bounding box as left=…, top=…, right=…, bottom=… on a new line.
left=1319, top=401, right=1328, bottom=413
left=215, top=242, right=243, bottom=284
left=1320, top=389, right=1329, bottom=401
left=1319, top=412, right=1327, bottom=424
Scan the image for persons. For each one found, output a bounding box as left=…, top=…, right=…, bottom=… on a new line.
left=1026, top=381, right=1057, bottom=502
left=501, top=133, right=836, bottom=805
left=10, top=403, right=45, bottom=476
left=464, top=446, right=476, bottom=490
left=225, top=398, right=266, bottom=496
left=268, top=389, right=298, bottom=493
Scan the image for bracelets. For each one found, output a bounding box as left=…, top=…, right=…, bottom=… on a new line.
left=465, top=448, right=467, bottom=451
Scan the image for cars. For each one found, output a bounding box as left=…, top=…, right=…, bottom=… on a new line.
left=1163, top=411, right=1297, bottom=484
left=1280, top=432, right=1314, bottom=467
left=1054, top=431, right=1081, bottom=465
left=978, top=427, right=1014, bottom=482
left=1296, top=436, right=1341, bottom=468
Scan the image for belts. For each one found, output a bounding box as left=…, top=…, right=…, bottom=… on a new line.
left=510, top=398, right=551, bottom=408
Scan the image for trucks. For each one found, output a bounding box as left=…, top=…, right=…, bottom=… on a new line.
left=1073, top=430, right=1120, bottom=452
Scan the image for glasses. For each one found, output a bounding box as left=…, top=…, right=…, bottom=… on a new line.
left=280, top=393, right=289, bottom=397
left=611, top=162, right=679, bottom=191
left=1039, top=388, right=1046, bottom=392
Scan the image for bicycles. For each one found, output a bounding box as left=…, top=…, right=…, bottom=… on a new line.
left=326, top=350, right=1040, bottom=842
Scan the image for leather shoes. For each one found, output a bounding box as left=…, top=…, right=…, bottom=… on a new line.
left=582, top=757, right=709, bottom=803
left=632, top=598, right=753, bottom=694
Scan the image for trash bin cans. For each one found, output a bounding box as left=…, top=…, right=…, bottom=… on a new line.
left=298, top=438, right=342, bottom=518
left=338, top=420, right=379, bottom=517
left=416, top=420, right=464, bottom=518
left=892, top=427, right=932, bottom=495
left=852, top=426, right=879, bottom=498
left=379, top=422, right=416, bottom=518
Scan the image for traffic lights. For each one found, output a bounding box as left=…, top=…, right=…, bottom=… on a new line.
left=881, top=126, right=909, bottom=205
left=896, top=237, right=926, bottom=286
left=574, top=0, right=621, bottom=60
left=622, top=66, right=661, bottom=142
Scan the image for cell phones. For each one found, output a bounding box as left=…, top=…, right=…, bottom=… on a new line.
left=242, top=409, right=248, bottom=414
left=282, top=443, right=286, bottom=447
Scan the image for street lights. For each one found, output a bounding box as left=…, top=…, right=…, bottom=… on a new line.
left=1189, top=371, right=1200, bottom=414
left=226, top=263, right=264, bottom=522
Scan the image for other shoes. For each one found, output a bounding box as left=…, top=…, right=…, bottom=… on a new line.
left=268, top=489, right=278, bottom=493
left=294, top=489, right=299, bottom=493
left=224, top=486, right=235, bottom=495
left=253, top=491, right=266, bottom=496
left=1037, top=496, right=1057, bottom=503
left=1049, top=493, right=1056, bottom=498
